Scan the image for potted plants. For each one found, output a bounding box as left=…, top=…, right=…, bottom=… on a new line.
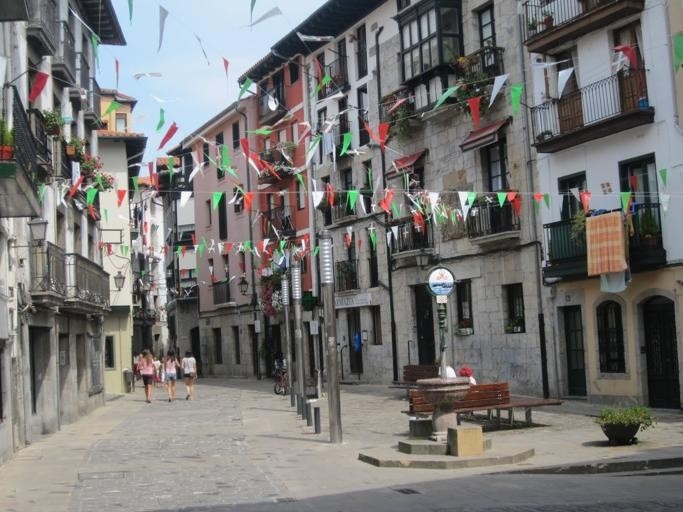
left=593, top=403, right=652, bottom=444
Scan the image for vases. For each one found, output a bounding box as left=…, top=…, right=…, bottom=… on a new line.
left=65, top=145, right=75, bottom=156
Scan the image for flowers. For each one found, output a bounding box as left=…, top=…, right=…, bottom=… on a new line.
left=68, top=136, right=114, bottom=191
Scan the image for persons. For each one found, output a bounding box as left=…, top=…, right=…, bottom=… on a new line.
left=165, top=349, right=179, bottom=400
left=132, top=352, right=142, bottom=380
left=135, top=348, right=160, bottom=405
left=151, top=356, right=166, bottom=386
left=181, top=350, right=198, bottom=400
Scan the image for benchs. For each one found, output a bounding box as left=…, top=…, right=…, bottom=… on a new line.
left=405, top=382, right=562, bottom=428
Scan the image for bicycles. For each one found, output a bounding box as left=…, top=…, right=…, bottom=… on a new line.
left=273, top=366, right=286, bottom=395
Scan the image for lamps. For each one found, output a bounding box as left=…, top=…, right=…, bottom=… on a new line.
left=110, top=270, right=126, bottom=294
left=237, top=276, right=253, bottom=296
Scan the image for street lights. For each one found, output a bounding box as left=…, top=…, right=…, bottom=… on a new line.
left=319, top=229, right=342, bottom=444
left=280, top=261, right=303, bottom=395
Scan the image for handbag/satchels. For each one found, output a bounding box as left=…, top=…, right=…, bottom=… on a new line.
left=190, top=372, right=197, bottom=378
